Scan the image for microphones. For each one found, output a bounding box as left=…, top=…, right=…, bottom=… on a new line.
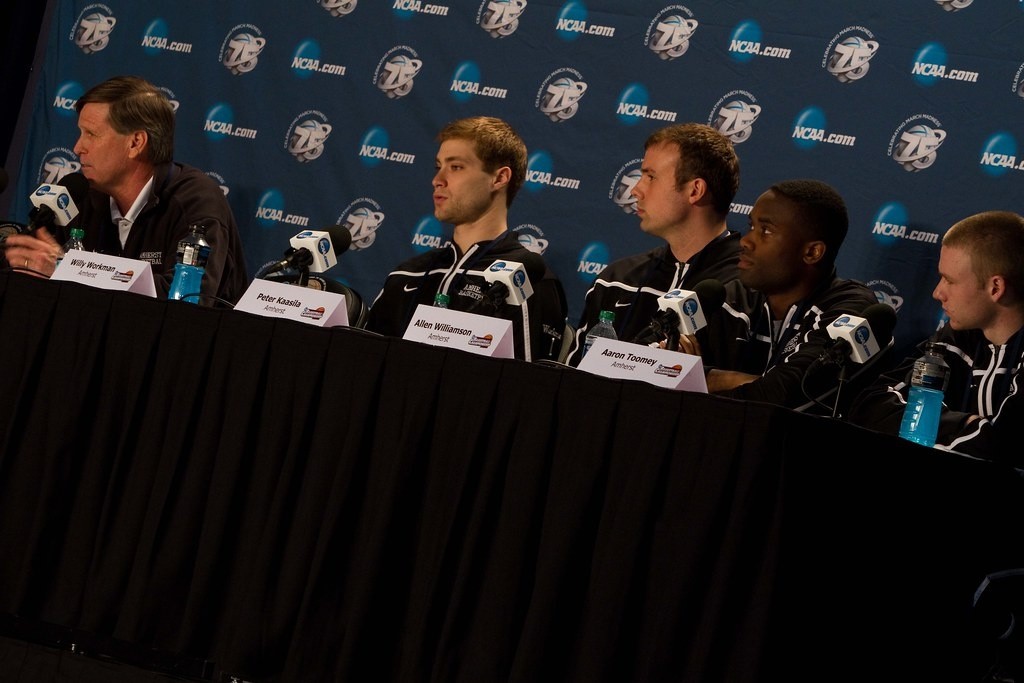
left=20, top=172, right=90, bottom=236
left=635, top=279, right=728, bottom=341
left=269, top=226, right=352, bottom=275
left=818, top=303, right=898, bottom=365
left=470, top=252, right=548, bottom=313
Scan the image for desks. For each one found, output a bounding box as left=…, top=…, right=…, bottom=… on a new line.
left=0, top=270, right=1024, bottom=683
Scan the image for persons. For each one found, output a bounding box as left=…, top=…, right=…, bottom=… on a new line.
left=559, top=121, right=742, bottom=369
left=846, top=209, right=1024, bottom=470
left=637, top=179, right=879, bottom=409
left=4, top=76, right=248, bottom=310
left=363, top=115, right=568, bottom=367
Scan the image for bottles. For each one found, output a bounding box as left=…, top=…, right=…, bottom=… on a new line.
left=581, top=309, right=619, bottom=368
left=168, top=224, right=210, bottom=305
left=56, top=228, right=85, bottom=274
left=898, top=342, right=951, bottom=448
left=431, top=293, right=449, bottom=310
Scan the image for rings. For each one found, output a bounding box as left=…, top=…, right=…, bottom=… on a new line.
left=25, top=259, right=28, bottom=269
